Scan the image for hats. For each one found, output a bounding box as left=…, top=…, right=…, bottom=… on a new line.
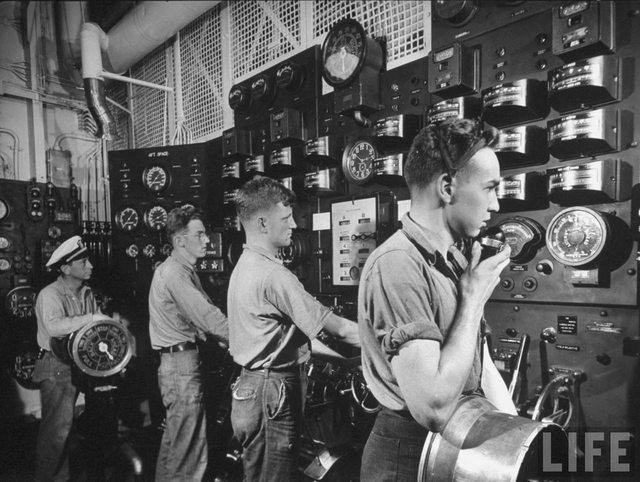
left=45, top=235, right=92, bottom=268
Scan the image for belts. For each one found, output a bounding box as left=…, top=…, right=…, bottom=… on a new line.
left=259, top=366, right=300, bottom=373
left=161, top=343, right=196, bottom=353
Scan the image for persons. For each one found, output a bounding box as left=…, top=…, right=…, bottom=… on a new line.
left=147, top=206, right=233, bottom=482
left=355, top=118, right=520, bottom=480
left=224, top=178, right=364, bottom=480
left=34, top=235, right=130, bottom=480
